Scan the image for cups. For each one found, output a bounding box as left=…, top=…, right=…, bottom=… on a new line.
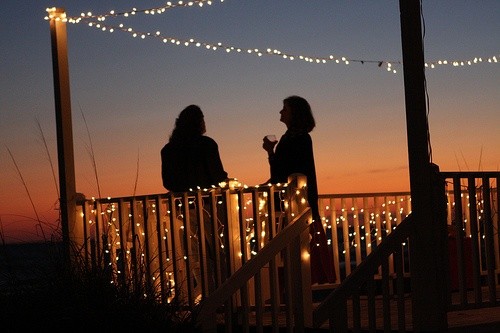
left=266, top=135, right=276, bottom=142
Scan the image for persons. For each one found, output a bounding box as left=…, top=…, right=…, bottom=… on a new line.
left=161, top=105, right=227, bottom=297
left=259, top=96, right=336, bottom=304
